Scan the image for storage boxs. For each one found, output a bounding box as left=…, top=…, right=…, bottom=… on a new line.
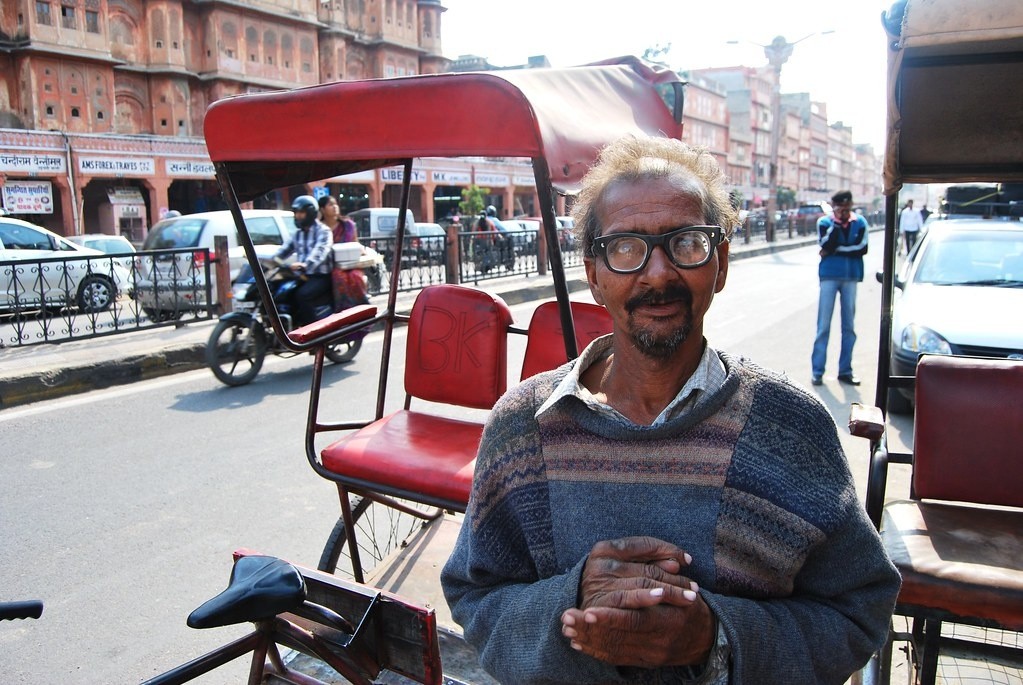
left=333, top=242, right=374, bottom=270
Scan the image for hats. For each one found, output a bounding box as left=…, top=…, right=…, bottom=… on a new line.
left=832, top=191, right=854, bottom=207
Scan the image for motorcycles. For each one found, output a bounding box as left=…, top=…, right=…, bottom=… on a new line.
left=206, top=254, right=373, bottom=387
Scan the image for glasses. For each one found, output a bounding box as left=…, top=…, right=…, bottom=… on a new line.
left=590, top=225, right=725, bottom=274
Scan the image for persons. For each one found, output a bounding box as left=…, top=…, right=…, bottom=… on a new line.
left=920, top=203, right=932, bottom=222
left=318, top=195, right=372, bottom=312
left=810, top=189, right=869, bottom=386
left=438, top=135, right=901, bottom=685
left=898, top=199, right=923, bottom=255
left=266, top=195, right=334, bottom=355
left=472, top=204, right=511, bottom=263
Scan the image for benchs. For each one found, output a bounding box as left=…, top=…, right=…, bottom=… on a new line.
left=847, top=354, right=1023, bottom=633
left=288, top=283, right=615, bottom=503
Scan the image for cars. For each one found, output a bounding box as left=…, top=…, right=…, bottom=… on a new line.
left=63, top=193, right=887, bottom=301
left=876, top=211, right=1023, bottom=417
left=0, top=218, right=134, bottom=319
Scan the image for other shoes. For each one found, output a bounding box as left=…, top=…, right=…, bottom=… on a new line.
left=837, top=374, right=860, bottom=386
left=812, top=375, right=823, bottom=385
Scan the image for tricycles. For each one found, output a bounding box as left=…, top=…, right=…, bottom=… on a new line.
left=0, top=53, right=684, bottom=685
left=849, top=1, right=1022, bottom=684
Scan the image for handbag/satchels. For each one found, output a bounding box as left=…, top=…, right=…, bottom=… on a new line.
left=335, top=253, right=373, bottom=270
left=331, top=240, right=361, bottom=262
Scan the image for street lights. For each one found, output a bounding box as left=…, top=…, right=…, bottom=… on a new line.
left=725, top=28, right=838, bottom=238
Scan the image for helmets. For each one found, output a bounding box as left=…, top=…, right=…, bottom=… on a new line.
left=291, top=195, right=319, bottom=229
left=487, top=205, right=498, bottom=216
